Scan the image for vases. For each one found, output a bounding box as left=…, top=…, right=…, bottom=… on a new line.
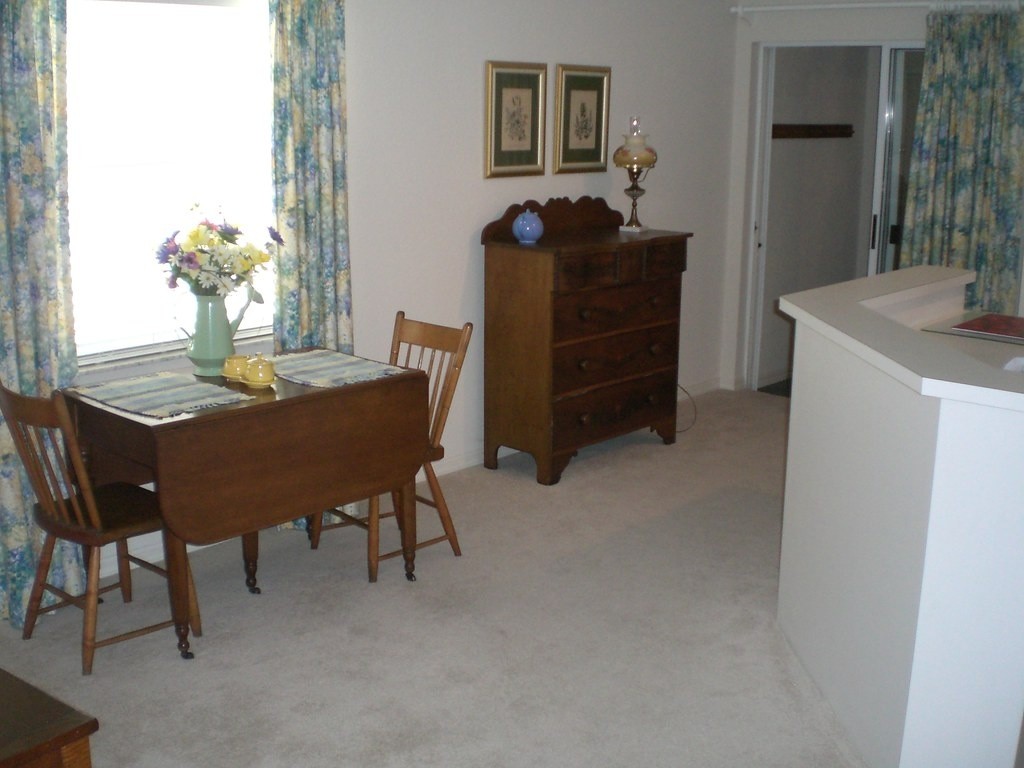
left=185, top=288, right=264, bottom=377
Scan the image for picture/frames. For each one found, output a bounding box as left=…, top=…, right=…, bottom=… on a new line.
left=484, top=61, right=547, bottom=178
left=551, top=64, right=612, bottom=175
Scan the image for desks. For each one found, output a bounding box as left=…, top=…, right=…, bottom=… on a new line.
left=63, top=347, right=428, bottom=660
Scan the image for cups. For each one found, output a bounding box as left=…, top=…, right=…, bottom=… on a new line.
left=245, top=352, right=275, bottom=383
left=224, top=354, right=251, bottom=376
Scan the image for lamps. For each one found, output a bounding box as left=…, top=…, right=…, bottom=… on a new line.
left=614, top=114, right=657, bottom=233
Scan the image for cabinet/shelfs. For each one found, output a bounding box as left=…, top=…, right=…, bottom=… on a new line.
left=483, top=228, right=693, bottom=486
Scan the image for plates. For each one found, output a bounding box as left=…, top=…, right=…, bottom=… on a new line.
left=239, top=377, right=276, bottom=388
left=221, top=372, right=242, bottom=382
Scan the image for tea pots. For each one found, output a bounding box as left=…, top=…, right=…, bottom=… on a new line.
left=512, top=208, right=544, bottom=245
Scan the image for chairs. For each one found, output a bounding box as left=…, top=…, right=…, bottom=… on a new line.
left=0, top=381, right=202, bottom=675
left=311, top=312, right=473, bottom=583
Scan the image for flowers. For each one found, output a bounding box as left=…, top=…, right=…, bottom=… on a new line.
left=154, top=218, right=286, bottom=296
